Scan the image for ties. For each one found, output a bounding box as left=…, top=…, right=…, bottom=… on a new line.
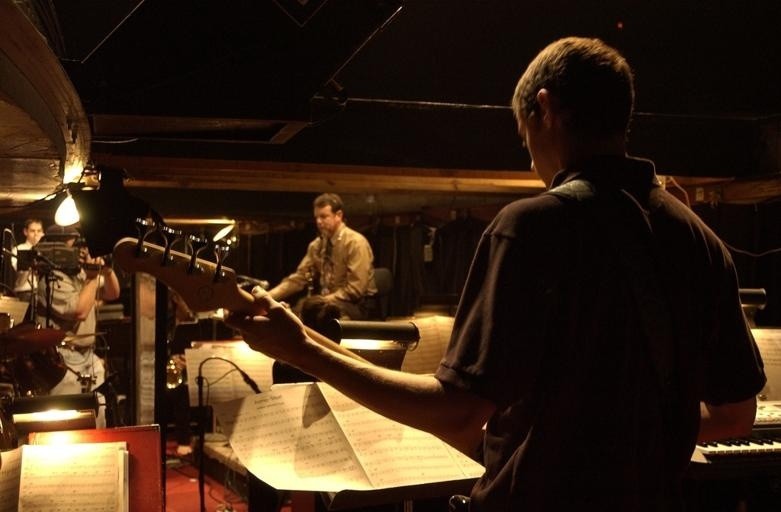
left=325, top=239, right=332, bottom=262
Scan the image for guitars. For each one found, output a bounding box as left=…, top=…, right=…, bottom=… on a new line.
left=111, top=215, right=486, bottom=469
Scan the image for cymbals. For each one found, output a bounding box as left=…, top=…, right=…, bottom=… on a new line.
left=62, top=332, right=110, bottom=344
left=4, top=326, right=66, bottom=353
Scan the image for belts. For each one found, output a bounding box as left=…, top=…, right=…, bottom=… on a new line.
left=57, top=341, right=91, bottom=355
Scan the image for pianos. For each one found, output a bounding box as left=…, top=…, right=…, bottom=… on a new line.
left=691, top=418, right=781, bottom=466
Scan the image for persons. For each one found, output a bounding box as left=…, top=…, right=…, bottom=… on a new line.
left=32, top=224, right=121, bottom=401
left=10, top=216, right=47, bottom=291
left=223, top=34, right=769, bottom=511
left=264, top=188, right=377, bottom=384
